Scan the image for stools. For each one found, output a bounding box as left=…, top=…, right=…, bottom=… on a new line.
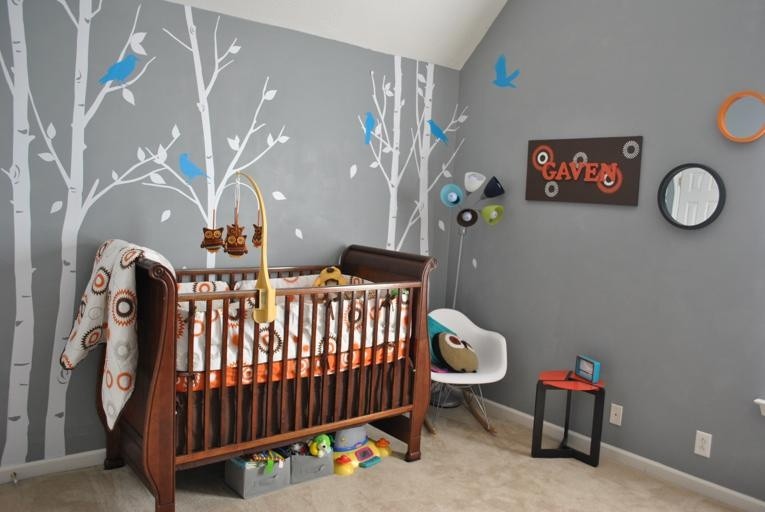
left=531, top=370, right=606, bottom=467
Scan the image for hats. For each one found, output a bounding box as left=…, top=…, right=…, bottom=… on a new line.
left=313, top=266, right=347, bottom=286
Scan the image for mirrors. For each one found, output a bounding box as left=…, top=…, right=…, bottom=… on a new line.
left=657, top=163, right=726, bottom=229
left=718, top=93, right=765, bottom=143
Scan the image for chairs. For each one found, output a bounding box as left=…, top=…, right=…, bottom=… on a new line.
left=423, top=308, right=508, bottom=435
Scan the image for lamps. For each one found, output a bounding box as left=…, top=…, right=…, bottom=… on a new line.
left=456, top=207, right=480, bottom=228
left=476, top=204, right=505, bottom=226
left=441, top=184, right=464, bottom=208
left=463, top=171, right=486, bottom=198
left=473, top=176, right=505, bottom=209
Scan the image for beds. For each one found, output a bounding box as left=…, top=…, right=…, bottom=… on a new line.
left=96, top=243, right=440, bottom=512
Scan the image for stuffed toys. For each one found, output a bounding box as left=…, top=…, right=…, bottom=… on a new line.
left=292, top=436, right=334, bottom=458
